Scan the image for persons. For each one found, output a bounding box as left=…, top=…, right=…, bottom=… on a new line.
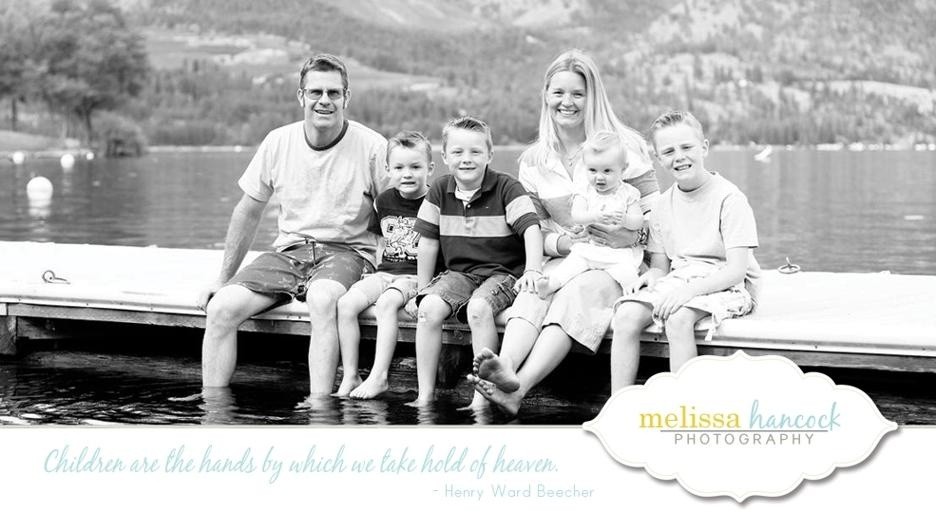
left=167, top=51, right=758, bottom=415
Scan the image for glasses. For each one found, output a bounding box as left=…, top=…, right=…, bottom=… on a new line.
left=301, top=87, right=346, bottom=100
